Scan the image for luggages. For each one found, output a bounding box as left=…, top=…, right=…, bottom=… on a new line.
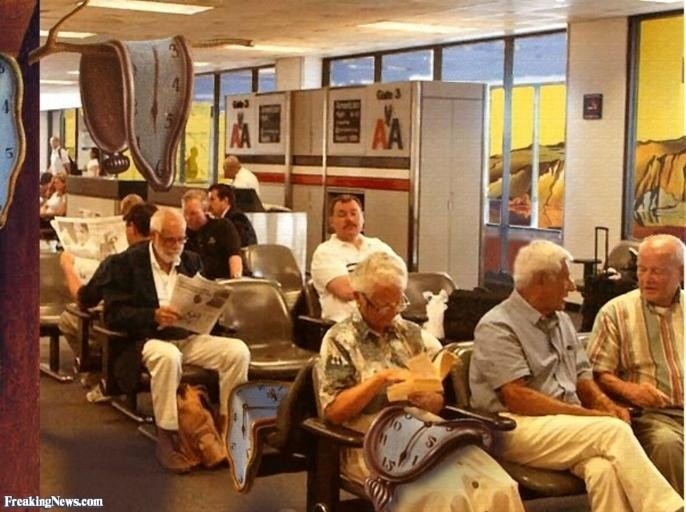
left=581, top=226, right=625, bottom=330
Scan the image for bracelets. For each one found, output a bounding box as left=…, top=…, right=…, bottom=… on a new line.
left=590, top=393, right=606, bottom=408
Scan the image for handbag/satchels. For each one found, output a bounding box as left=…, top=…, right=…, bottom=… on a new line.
left=444, top=288, right=493, bottom=342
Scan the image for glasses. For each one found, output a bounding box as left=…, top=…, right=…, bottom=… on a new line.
left=152, top=231, right=189, bottom=246
left=362, top=292, right=410, bottom=314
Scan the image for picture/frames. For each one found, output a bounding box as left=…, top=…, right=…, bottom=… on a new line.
left=616, top=6, right=685, bottom=244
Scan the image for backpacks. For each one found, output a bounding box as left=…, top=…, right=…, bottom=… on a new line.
left=178, top=384, right=226, bottom=468
left=58, top=148, right=82, bottom=175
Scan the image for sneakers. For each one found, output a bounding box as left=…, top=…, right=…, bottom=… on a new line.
left=87, top=383, right=109, bottom=402
left=157, top=442, right=193, bottom=475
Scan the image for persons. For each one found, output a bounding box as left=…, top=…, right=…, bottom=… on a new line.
left=585, top=233, right=684, bottom=497
left=469, top=238, right=684, bottom=512
left=309, top=195, right=407, bottom=325
left=316, top=252, right=526, bottom=512
left=38, top=135, right=261, bottom=402
left=104, top=208, right=251, bottom=472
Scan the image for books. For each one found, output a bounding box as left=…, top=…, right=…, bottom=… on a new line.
left=385, top=346, right=465, bottom=403
